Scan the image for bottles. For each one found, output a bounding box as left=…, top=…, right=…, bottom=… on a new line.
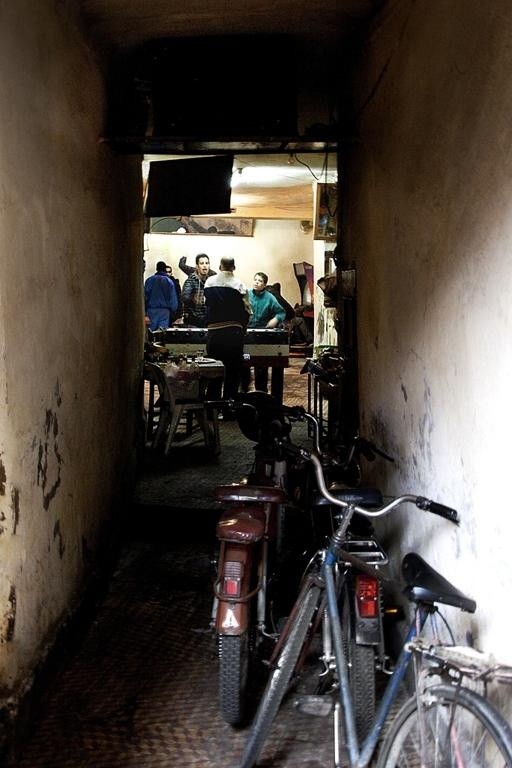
left=164, top=355, right=201, bottom=400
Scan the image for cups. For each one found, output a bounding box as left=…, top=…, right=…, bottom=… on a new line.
left=197, top=352, right=203, bottom=361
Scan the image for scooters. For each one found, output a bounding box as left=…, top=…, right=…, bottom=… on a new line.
left=194, top=389, right=327, bottom=726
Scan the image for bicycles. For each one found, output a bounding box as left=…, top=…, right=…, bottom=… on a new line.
left=278, top=404, right=400, bottom=754
left=241, top=437, right=510, bottom=766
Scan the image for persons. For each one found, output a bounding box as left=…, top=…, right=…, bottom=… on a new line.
left=143, top=253, right=287, bottom=419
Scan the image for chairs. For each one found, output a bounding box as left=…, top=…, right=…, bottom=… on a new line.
left=150, top=363, right=222, bottom=456
